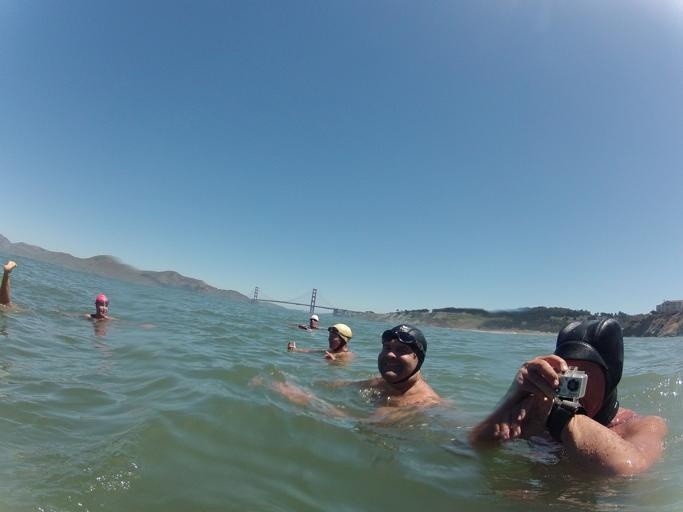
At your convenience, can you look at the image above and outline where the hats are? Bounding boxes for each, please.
[379,323,428,365]
[551,316,626,394]
[328,323,352,343]
[310,314,319,321]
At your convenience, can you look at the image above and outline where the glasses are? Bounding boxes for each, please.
[381,330,415,344]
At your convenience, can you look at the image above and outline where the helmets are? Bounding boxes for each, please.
[94,294,111,307]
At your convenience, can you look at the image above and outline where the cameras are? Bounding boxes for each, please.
[544,372,588,398]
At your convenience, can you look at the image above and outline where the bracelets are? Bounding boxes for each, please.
[544,399,587,441]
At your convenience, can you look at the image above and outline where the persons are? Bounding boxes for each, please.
[464,315,668,482]
[287,322,356,363]
[0,259,18,310]
[52,291,155,329]
[252,323,442,428]
[297,314,321,332]
